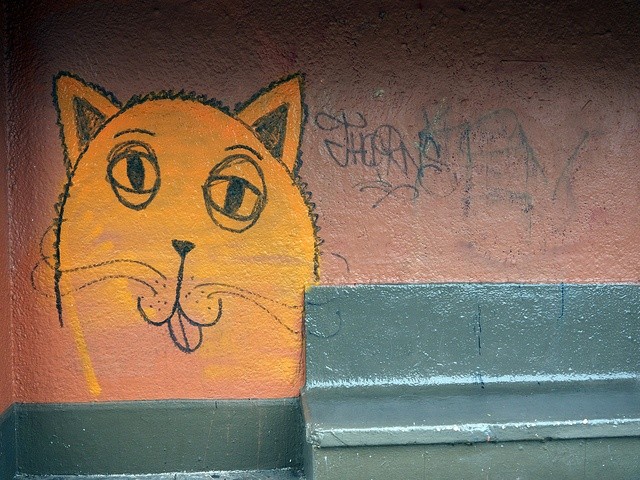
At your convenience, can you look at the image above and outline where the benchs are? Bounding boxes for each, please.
[300,281,639,476]
